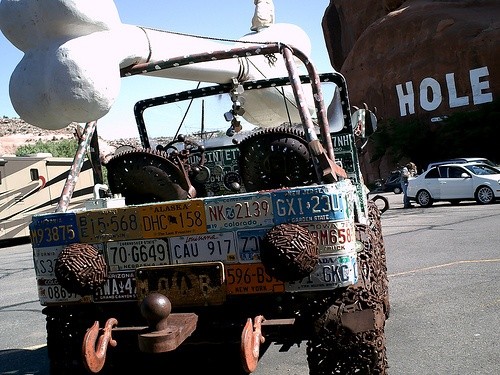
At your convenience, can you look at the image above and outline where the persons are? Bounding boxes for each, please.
[409,165,418,178]
[401,162,415,208]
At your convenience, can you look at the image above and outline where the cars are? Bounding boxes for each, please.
[407,162,500,207]
[384,177,408,194]
[447,158,496,167]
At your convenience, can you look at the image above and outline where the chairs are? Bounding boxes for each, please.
[236,125,325,193]
[106,149,189,205]
